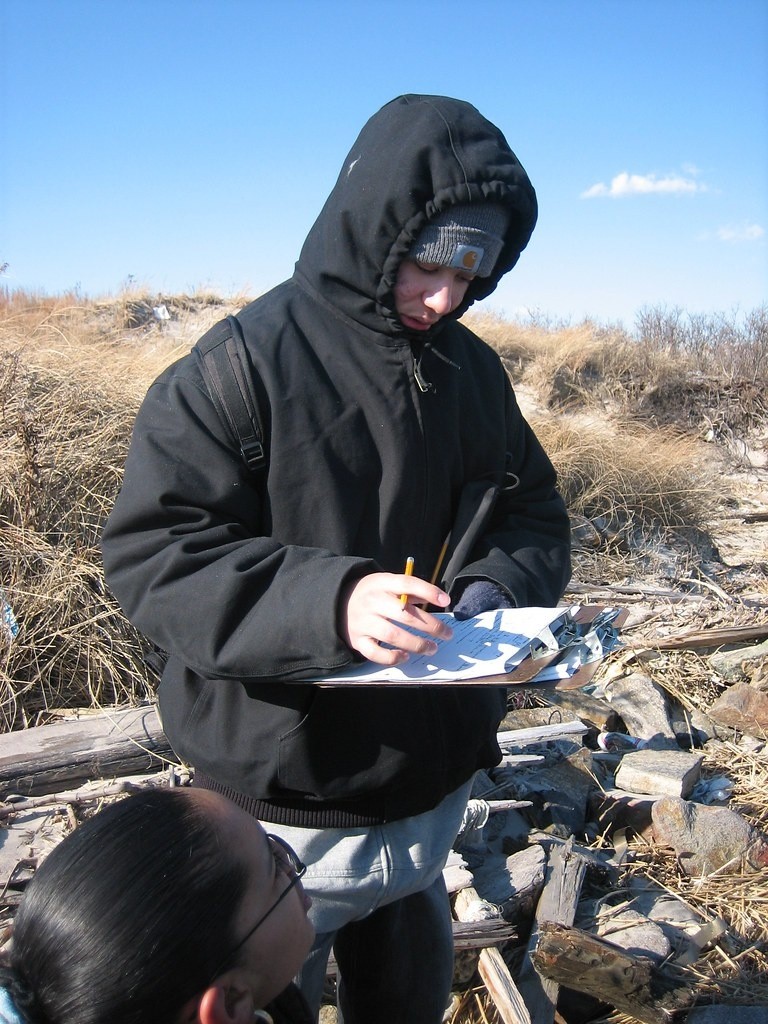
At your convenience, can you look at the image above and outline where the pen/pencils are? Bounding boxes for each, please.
[400,557,414,611]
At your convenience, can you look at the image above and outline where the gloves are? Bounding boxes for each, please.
[453,580,514,621]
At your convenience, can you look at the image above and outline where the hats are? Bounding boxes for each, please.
[408,200,512,278]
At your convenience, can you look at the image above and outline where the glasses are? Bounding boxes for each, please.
[187,832,307,1024]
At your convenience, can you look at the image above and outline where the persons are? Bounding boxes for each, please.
[97,91,576,1023]
[0,787,321,1024]
